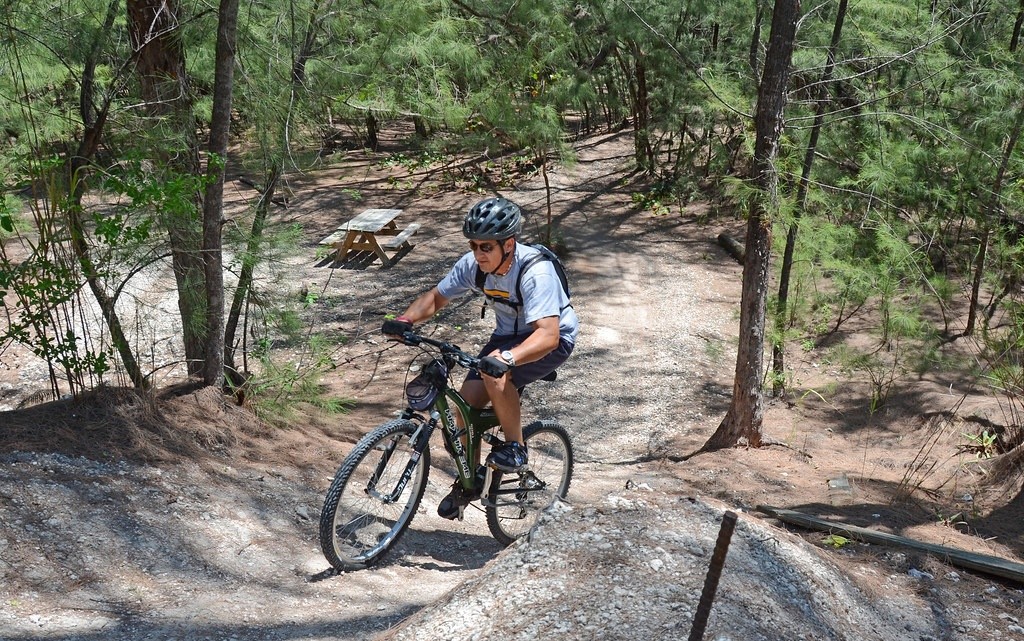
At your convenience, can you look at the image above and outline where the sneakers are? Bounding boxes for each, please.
[438,475,472,519]
[486,441,529,472]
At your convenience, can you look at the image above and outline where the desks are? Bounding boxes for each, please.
[335,209,411,267]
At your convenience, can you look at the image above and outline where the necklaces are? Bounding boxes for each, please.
[502,256,514,276]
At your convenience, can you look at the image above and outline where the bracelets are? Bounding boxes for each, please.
[396,317,413,325]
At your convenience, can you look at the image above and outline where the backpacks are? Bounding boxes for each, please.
[475,242,573,334]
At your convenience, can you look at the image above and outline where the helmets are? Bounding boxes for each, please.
[463,198,521,240]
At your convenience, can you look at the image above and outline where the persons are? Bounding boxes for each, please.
[384,199,580,518]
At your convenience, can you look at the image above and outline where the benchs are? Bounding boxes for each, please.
[318,231,347,246]
[383,223,421,249]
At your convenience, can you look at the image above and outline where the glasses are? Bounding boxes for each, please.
[468,240,498,252]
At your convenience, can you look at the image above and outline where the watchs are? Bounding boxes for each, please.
[497,351,514,366]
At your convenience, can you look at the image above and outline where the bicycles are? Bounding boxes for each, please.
[320,327,576,573]
